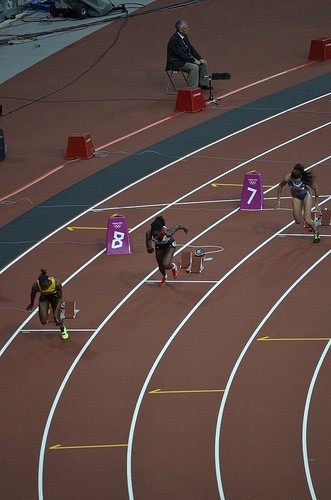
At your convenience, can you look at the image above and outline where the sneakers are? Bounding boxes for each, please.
[160,274,168,287]
[61,325,68,340]
[313,229,320,243]
[171,262,178,279]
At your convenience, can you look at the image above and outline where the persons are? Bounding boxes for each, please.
[167,20,214,90]
[276,164,320,243]
[145,216,188,288]
[26,269,69,339]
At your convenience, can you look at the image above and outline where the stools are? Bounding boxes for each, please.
[165,68,189,95]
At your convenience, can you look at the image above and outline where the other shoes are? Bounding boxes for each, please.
[198,84,213,90]
